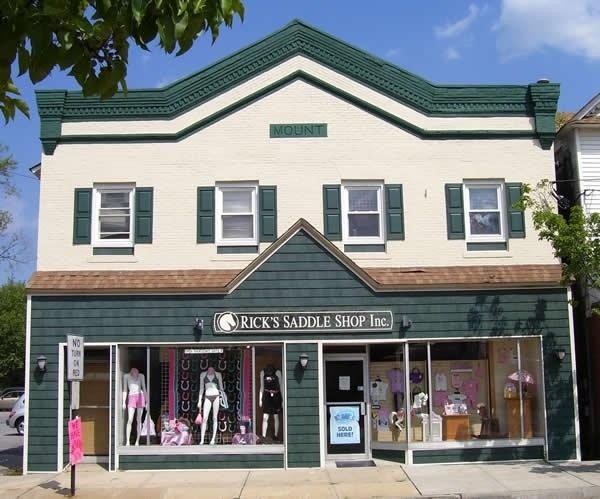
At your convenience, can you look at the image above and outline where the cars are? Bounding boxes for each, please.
[0,387,25,435]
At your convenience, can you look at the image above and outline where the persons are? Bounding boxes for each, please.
[259,366,283,438]
[197,367,229,445]
[122,368,148,446]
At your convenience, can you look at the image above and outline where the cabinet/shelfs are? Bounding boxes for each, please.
[442,414,471,440]
[504,396,534,438]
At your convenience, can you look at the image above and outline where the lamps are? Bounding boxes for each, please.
[552,345,566,361]
[298,353,310,369]
[37,354,48,372]
